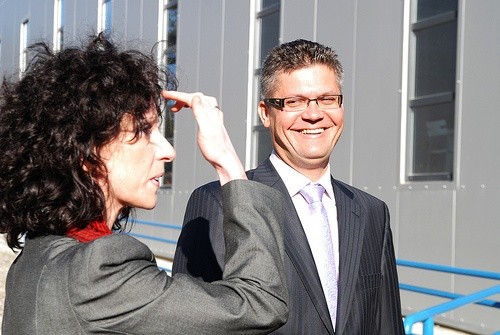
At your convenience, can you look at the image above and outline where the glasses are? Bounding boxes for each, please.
[263,95,343,111]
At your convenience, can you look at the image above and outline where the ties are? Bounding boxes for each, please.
[300,185,337,332]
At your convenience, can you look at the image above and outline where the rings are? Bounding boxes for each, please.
[215,106,218,108]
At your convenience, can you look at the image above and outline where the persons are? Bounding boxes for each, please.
[171,39,406,335]
[0,30,289,335]
